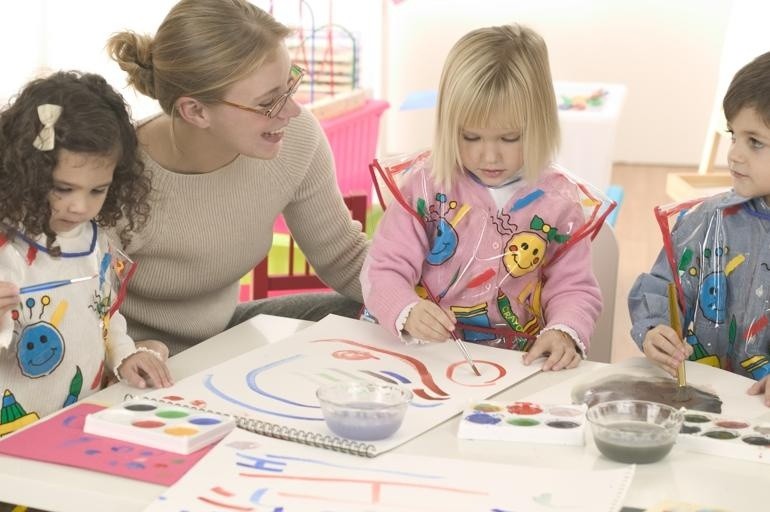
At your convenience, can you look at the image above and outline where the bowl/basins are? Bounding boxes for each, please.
[316,380,414,441]
[585,399,685,464]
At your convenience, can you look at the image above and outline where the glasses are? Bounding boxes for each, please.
[215,64,305,120]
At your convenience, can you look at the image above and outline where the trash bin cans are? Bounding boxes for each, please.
[535,80,621,212]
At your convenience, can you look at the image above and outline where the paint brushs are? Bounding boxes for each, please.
[421,278,482,378]
[19,274,101,296]
[669,283,694,400]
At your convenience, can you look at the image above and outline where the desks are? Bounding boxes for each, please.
[0,309,769,510]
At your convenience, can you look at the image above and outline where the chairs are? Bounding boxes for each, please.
[247,98,390,306]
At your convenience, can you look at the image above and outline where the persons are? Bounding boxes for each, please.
[627,51,770,408]
[0,70,174,438]
[358,21,617,371]
[105,1,371,358]
